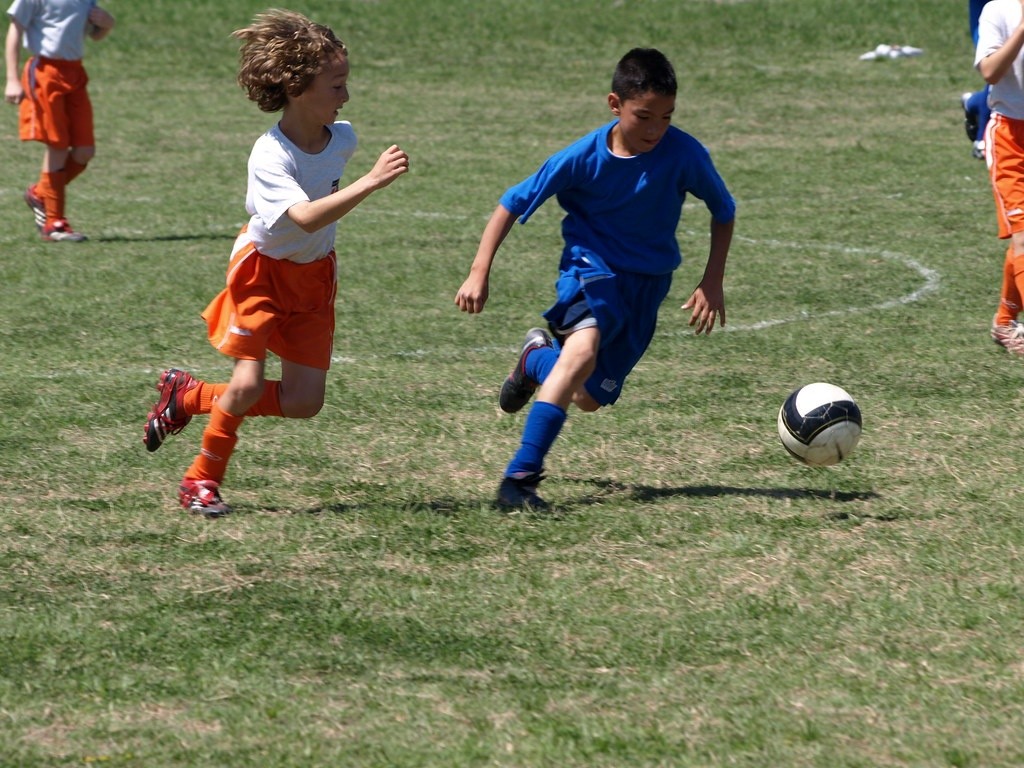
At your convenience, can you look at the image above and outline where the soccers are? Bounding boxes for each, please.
[776,381,863,469]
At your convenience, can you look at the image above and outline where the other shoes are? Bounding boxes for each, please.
[972,140,986,159]
[961,92,979,142]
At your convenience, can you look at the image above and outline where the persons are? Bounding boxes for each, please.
[5,1,115,241]
[142,9,410,515]
[452,49,737,512]
[960,0,1024,357]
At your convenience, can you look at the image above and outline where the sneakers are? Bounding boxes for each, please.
[42,217,87,244]
[991,313,1024,358]
[491,474,552,513]
[144,368,199,452]
[500,326,554,413]
[179,476,233,517]
[22,184,47,232]
